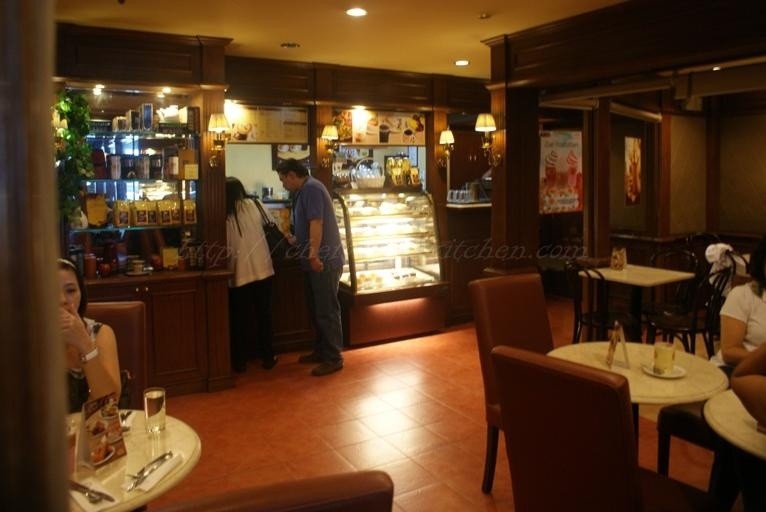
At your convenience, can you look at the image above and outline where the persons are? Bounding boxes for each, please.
[57,258,122,414]
[275,157,346,376]
[225,176,284,373]
[730,340,766,427]
[708,241,766,390]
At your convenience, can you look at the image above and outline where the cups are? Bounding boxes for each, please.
[380,125,391,142]
[567,164,578,186]
[142,386,165,432]
[545,166,557,189]
[261,185,273,202]
[653,337,676,373]
[403,128,417,145]
[273,192,290,202]
[131,259,146,273]
[445,188,475,203]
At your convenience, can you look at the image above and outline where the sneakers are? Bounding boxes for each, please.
[312,361,343,376]
[299,354,322,364]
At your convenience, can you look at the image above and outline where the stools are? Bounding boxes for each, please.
[653,396,718,483]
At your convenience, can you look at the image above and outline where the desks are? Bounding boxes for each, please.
[55,405,205,512]
[702,387,765,465]
[585,259,698,344]
[543,340,728,448]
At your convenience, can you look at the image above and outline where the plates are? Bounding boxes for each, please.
[89,401,121,467]
[126,270,148,277]
[382,115,408,133]
[638,363,688,379]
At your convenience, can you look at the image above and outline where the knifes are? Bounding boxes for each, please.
[68,478,115,501]
[125,453,173,492]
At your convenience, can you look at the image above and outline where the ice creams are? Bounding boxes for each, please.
[545,150,559,183]
[566,149,579,185]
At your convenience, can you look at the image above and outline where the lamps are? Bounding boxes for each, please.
[433,128,459,174]
[203,111,231,170]
[315,123,340,175]
[472,111,504,170]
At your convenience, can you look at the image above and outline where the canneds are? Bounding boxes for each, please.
[132,260,144,273]
[84,253,97,280]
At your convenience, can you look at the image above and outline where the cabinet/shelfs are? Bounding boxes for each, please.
[326,187,452,353]
[69,122,201,232]
[77,264,232,393]
[256,201,319,352]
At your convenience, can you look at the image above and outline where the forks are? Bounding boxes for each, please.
[127,451,170,478]
[71,484,103,504]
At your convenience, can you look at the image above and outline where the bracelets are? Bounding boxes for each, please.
[81,348,98,363]
[308,255,316,263]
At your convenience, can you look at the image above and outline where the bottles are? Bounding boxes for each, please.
[610,246,628,272]
[108,142,179,181]
[69,246,96,279]
[178,228,203,272]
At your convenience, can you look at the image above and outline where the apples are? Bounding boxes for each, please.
[98,264,111,278]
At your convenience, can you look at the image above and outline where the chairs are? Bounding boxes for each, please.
[79,296,155,412]
[566,259,633,341]
[145,467,394,512]
[488,339,709,511]
[465,271,556,496]
[633,229,753,363]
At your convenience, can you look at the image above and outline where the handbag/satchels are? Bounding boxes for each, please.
[250,196,298,276]
